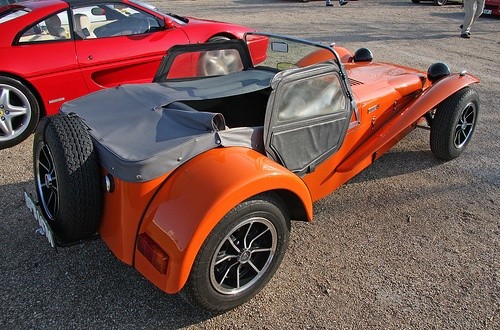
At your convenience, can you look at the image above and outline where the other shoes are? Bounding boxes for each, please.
[459,25,471,35]
[460,33,470,39]
[325,4,333,6]
[340,1,348,5]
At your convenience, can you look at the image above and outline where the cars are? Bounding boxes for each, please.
[412,0,500,19]
[0,0,268,148]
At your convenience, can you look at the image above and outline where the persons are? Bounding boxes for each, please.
[459,0,485,39]
[326,0,348,6]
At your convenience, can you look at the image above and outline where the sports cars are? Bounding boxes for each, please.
[28,31,480,315]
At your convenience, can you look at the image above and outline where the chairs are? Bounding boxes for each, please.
[74,13,97,39]
[45,14,66,38]
